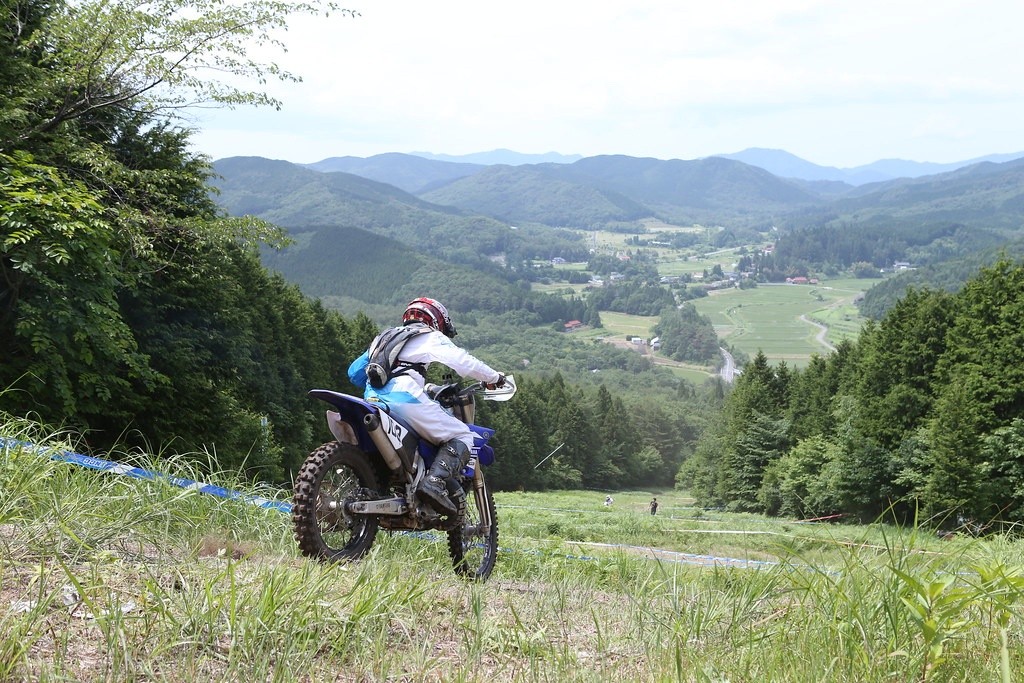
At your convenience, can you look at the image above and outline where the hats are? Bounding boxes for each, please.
[606,495,610,499]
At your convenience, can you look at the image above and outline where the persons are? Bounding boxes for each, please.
[348,297,505,515]
[604,496,613,506]
[648,498,658,515]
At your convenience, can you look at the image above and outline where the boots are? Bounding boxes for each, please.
[416,438,470,516]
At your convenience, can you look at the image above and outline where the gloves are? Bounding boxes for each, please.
[486,373,505,390]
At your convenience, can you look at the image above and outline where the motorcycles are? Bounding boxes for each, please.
[291,369,516,583]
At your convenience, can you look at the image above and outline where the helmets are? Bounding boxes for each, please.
[403,297,457,338]
